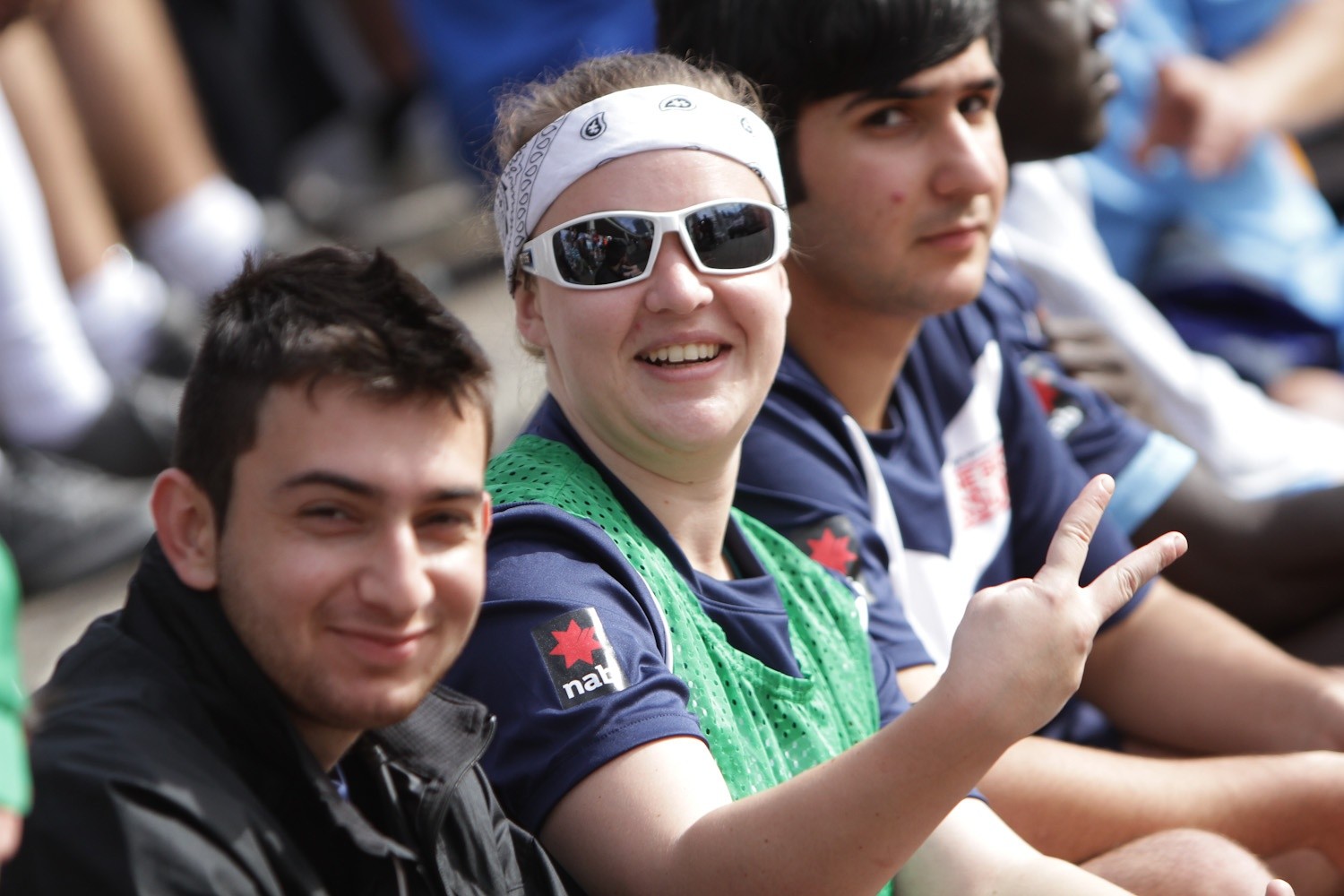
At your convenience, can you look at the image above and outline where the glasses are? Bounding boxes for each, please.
[514,200,792,290]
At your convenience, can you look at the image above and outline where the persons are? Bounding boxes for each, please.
[0,247,571,896]
[651,0,1344,896]
[0,0,1344,896]
[428,47,1187,896]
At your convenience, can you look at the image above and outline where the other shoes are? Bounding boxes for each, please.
[131,284,212,375]
[68,368,198,476]
[1,435,161,592]
